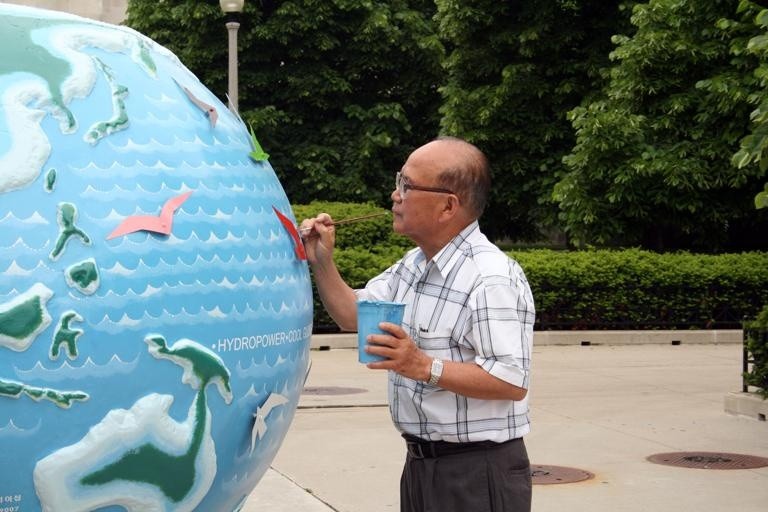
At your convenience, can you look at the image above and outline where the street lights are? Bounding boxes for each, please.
[217,0,245,112]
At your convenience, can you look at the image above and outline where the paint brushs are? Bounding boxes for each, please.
[297,213,386,232]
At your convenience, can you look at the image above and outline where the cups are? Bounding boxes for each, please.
[355,300,407,365]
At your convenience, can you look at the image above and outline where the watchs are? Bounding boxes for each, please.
[424,357,445,386]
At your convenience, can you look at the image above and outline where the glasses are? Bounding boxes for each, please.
[395,171,455,200]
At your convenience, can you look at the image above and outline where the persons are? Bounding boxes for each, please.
[299,135,537,511]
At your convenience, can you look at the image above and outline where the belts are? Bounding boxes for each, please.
[405,439,516,459]
